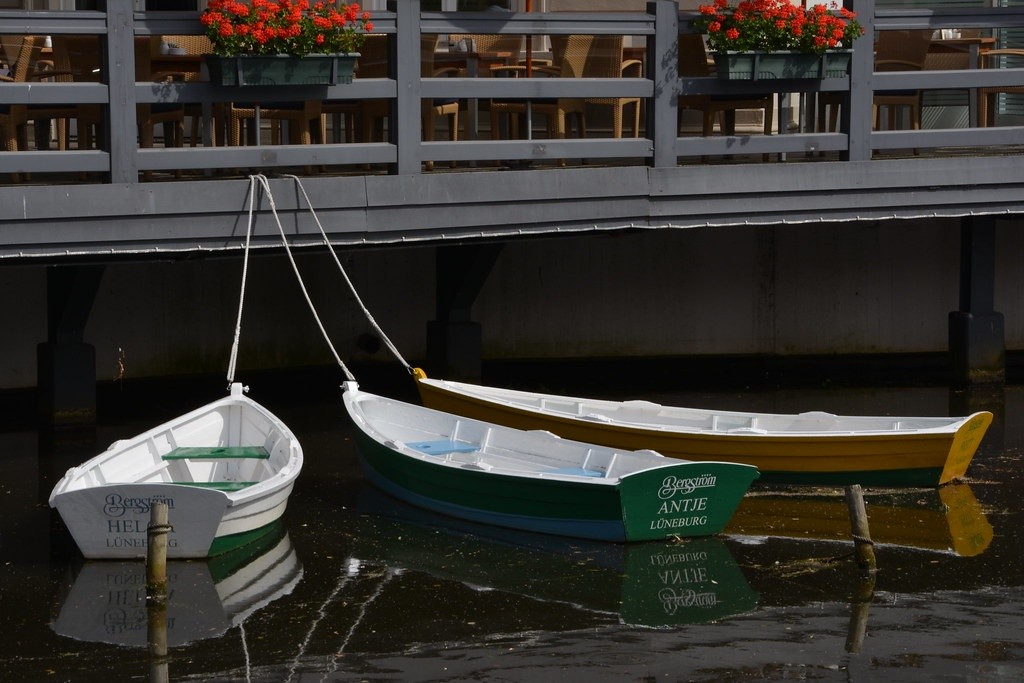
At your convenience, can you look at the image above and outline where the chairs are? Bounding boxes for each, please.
[0,30,1024,183]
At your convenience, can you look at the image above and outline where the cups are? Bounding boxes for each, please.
[160,42,170,54]
[941,28,952,39]
[952,29,961,39]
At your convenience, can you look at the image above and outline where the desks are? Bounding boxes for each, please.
[927,37,999,128]
[152,53,216,178]
[436,52,513,167]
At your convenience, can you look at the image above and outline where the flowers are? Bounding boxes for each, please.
[199,0,374,57]
[685,0,868,53]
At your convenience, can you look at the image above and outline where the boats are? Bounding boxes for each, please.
[415,367,994,485]
[49,380,305,557]
[721,472,995,559]
[346,501,761,629]
[53,514,306,647]
[342,379,761,544]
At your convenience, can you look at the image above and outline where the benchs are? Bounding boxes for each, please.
[540,466,605,477]
[161,447,268,461]
[168,482,259,491]
[405,441,478,455]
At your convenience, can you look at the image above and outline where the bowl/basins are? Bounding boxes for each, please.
[170,48,185,54]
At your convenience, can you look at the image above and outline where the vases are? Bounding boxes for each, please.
[707,47,856,81]
[216,52,362,86]
